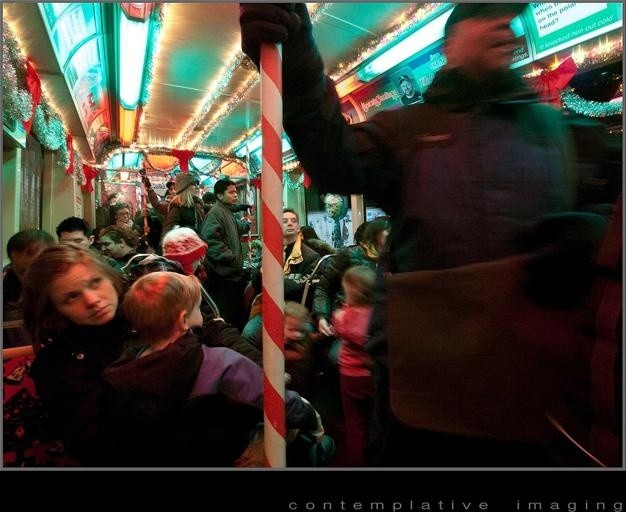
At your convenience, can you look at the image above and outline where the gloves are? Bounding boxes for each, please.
[238,2,329,84]
[510,203,616,314]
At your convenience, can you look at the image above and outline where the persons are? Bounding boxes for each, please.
[0,168,393,468]
[238,2,625,469]
[398,74,422,106]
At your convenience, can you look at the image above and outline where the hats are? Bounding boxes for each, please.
[399,74,411,83]
[444,3,529,28]
[176,173,199,194]
[162,224,208,278]
[166,177,176,187]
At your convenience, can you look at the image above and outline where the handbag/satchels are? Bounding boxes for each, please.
[377,249,623,451]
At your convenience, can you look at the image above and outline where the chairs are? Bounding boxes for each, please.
[301,253,338,313]
